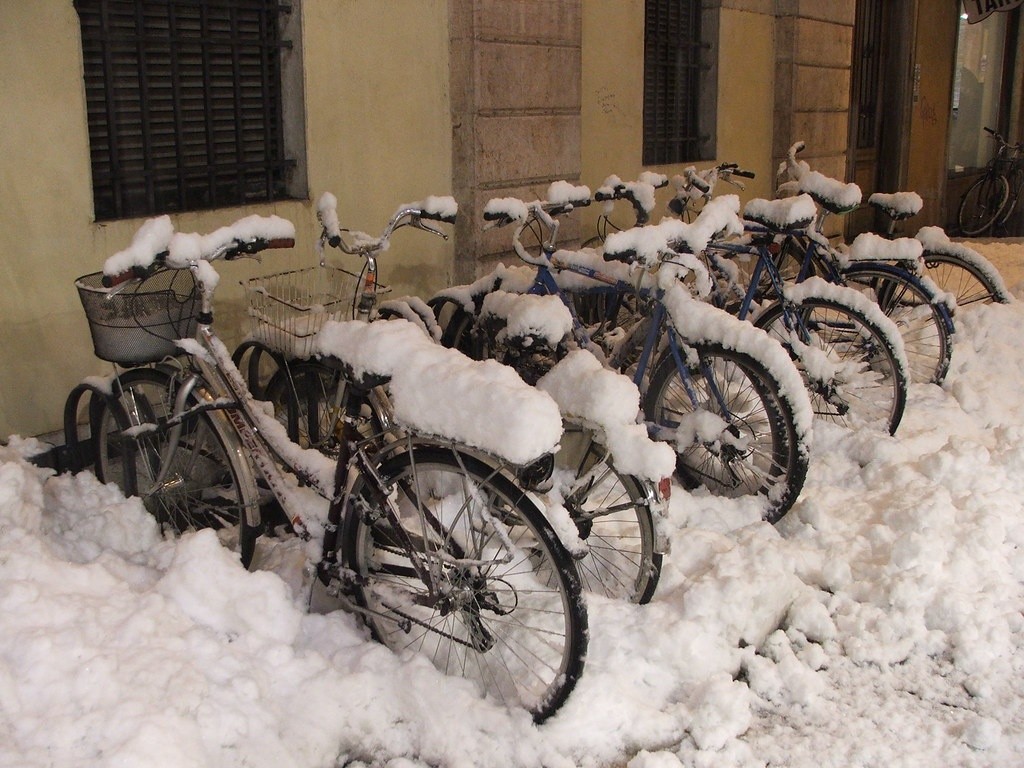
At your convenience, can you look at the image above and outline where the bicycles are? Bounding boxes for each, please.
[262,205,674,607]
[73,233,588,725]
[957,126,1024,238]
[413,136,1010,528]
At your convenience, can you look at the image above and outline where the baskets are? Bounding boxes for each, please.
[238,264,391,362]
[73,266,202,366]
